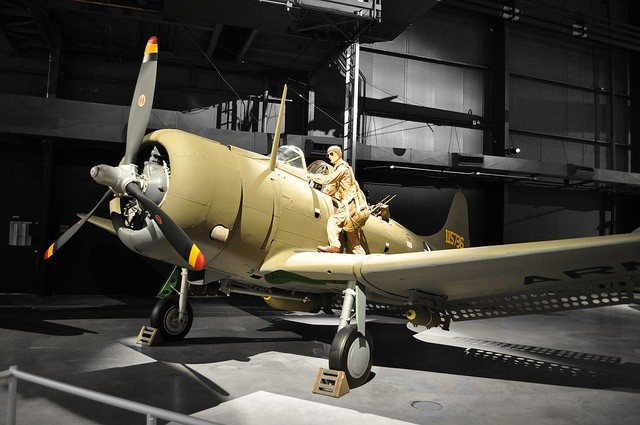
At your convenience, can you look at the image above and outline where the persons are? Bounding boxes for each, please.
[307,145,370,255]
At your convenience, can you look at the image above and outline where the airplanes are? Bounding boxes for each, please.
[43,35,640,388]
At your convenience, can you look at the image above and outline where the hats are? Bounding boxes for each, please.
[327,146,342,159]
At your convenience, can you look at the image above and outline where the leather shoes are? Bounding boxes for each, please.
[317,245,340,252]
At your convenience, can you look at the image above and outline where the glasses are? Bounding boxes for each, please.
[322,167,328,169]
[327,153,333,157]
